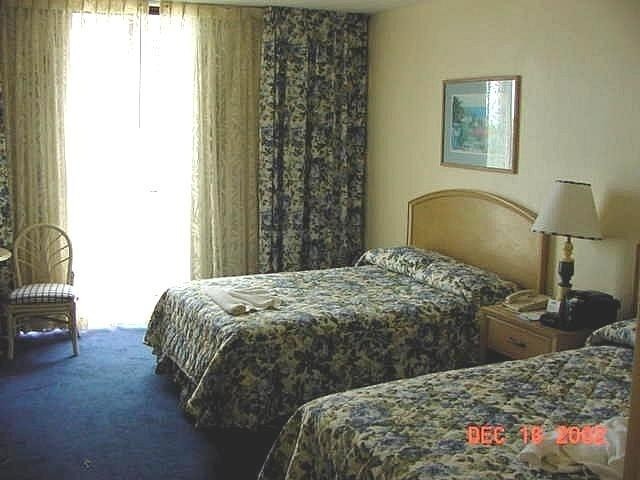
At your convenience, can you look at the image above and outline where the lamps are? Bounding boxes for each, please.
[531,178,604,312]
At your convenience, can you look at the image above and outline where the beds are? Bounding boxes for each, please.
[143,190,550,433]
[257,244,640,480]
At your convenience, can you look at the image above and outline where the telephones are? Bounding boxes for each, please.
[502,288,549,312]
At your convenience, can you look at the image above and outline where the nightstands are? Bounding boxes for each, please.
[480,304,593,366]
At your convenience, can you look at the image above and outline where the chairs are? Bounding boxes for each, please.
[8,224,80,361]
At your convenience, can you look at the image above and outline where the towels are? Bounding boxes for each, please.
[585,317,635,347]
[517,415,629,480]
[355,245,457,278]
[208,286,281,315]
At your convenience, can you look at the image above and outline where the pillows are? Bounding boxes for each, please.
[413,261,521,300]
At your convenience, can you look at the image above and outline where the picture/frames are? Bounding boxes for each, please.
[440,75,520,174]
